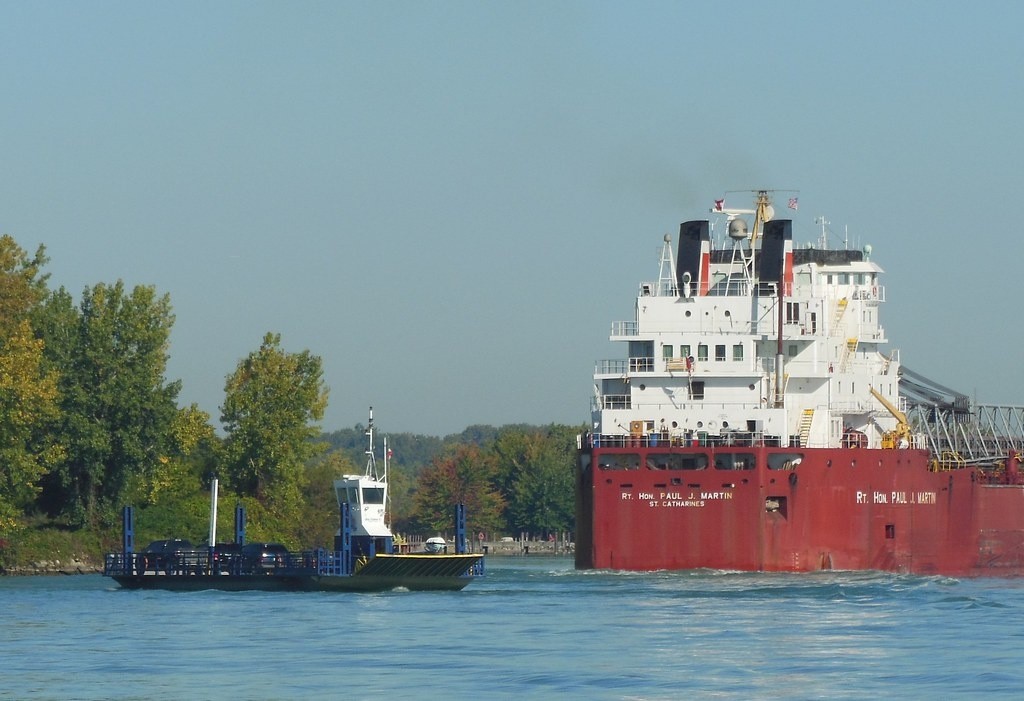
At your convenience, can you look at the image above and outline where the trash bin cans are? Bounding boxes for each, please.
[790,436,800,448]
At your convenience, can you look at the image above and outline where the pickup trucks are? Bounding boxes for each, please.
[135,539,207,574]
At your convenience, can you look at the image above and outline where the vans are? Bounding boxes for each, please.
[499,537,513,542]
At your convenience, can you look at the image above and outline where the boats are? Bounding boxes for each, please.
[574,188,1024,582]
[425,537,445,550]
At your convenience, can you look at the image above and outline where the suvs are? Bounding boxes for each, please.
[229,543,291,576]
[188,543,234,576]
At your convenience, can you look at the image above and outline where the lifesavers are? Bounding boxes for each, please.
[135,554,148,568]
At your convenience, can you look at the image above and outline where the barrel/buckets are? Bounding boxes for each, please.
[593,433,661,448]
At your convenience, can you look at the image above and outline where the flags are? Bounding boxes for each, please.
[788,197,798,210]
[714,198,725,212]
